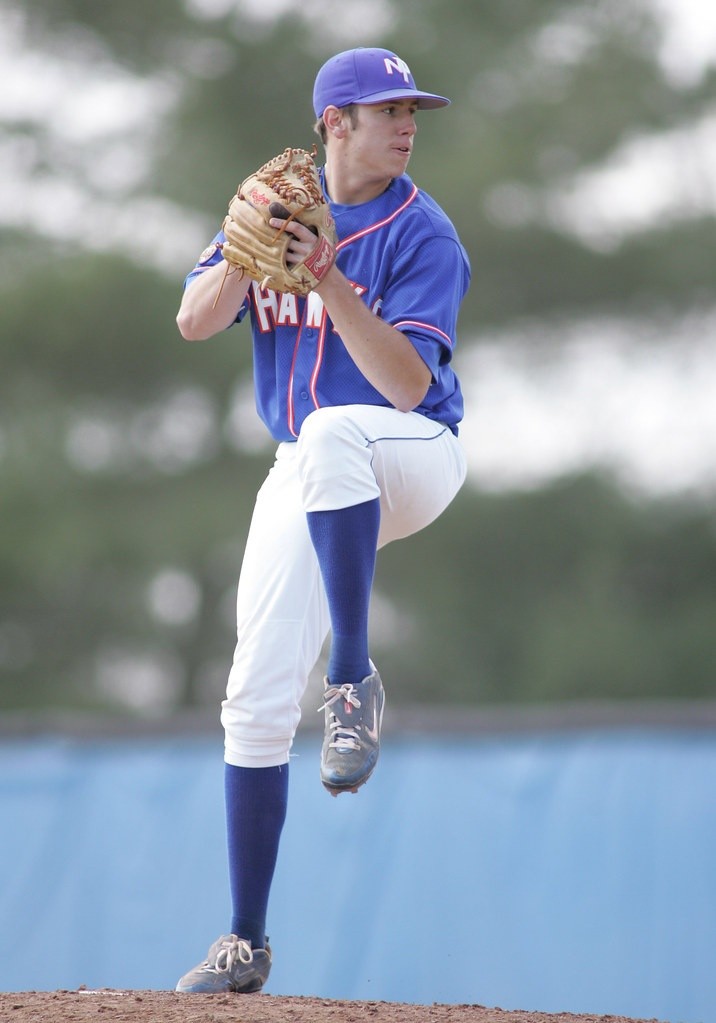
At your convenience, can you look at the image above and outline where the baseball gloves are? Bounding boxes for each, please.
[214,143,339,300]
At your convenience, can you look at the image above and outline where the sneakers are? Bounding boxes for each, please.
[176,935,273,997]
[316,657,385,795]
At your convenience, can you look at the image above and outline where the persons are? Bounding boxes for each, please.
[174,48,470,994]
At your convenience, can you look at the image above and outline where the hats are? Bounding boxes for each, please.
[312,48,450,120]
